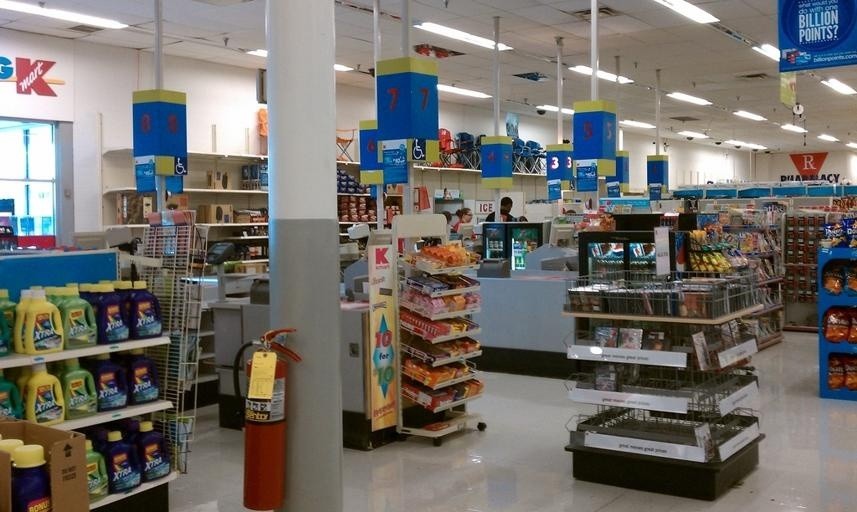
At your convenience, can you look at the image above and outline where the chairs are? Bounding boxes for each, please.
[332,127,357,162]
[427,128,547,174]
[254,110,267,153]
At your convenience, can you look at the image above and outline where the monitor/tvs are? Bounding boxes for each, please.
[364,230,392,252]
[458,223,473,236]
[550,224,574,244]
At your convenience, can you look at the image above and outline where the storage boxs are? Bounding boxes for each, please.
[162,195,190,212]
[205,168,231,191]
[0,417,94,512]
[199,202,235,225]
[235,262,268,273]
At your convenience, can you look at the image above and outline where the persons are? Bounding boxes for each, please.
[454,208,475,240]
[442,187,452,198]
[438,210,457,234]
[485,196,517,222]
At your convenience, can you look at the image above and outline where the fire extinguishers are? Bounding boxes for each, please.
[233,329,302,510]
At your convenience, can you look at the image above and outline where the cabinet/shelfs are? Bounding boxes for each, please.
[97,145,403,412]
[677,229,736,283]
[393,215,487,448]
[673,185,857,209]
[0,250,179,512]
[700,210,787,352]
[410,161,552,226]
[816,244,857,404]
[778,204,857,333]
[563,272,765,502]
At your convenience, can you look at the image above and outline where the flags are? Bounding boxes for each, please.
[777,71,796,108]
[777,0,857,75]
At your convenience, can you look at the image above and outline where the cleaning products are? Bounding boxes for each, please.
[1,421,173,512]
[1,346,174,425]
[1,278,173,357]
